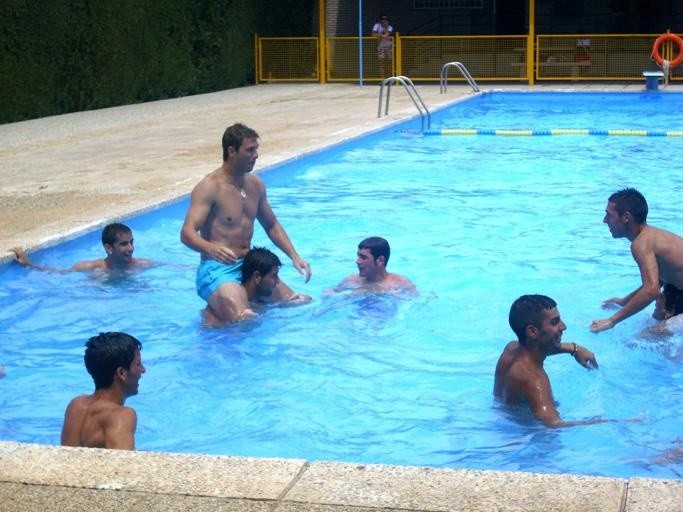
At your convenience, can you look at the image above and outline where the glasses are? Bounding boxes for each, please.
[380,18,388,20]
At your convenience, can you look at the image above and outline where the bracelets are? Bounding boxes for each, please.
[570,341,579,357]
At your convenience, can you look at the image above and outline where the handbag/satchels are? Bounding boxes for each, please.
[520,50,566,62]
[575,54,592,62]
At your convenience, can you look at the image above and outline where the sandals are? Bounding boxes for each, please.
[378,81,383,85]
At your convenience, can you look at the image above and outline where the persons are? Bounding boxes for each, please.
[590,183,681,337]
[199,244,315,327]
[371,12,394,86]
[629,284,682,363]
[4,222,196,282]
[321,235,420,299]
[58,332,147,452]
[179,121,310,325]
[621,442,682,470]
[491,293,642,432]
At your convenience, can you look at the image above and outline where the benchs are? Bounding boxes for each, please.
[510,47,592,77]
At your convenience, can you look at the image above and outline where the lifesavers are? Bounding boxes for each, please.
[652,34,683,68]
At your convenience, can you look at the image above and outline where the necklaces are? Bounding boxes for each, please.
[219,165,250,199]
